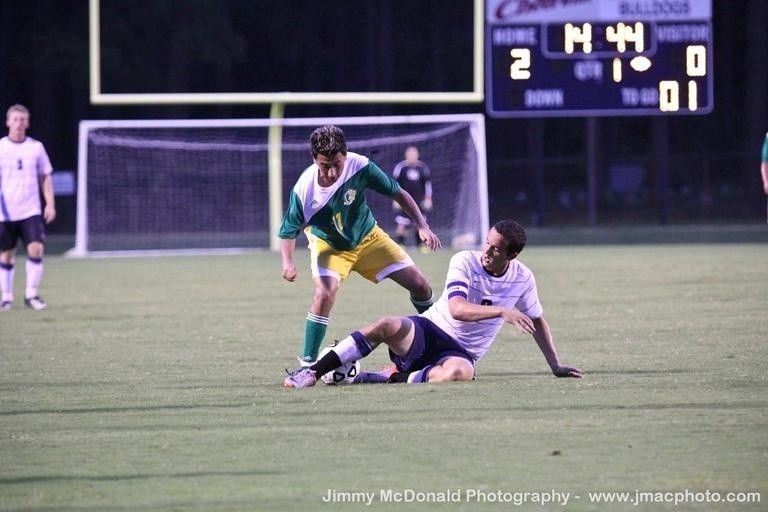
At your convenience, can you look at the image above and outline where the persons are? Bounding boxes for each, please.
[390,147,434,255]
[760,131,768,195]
[0,104,56,311]
[283,220,582,389]
[277,124,443,371]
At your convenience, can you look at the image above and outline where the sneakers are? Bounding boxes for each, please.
[284,369,315,388]
[354,370,369,384]
[1,298,49,314]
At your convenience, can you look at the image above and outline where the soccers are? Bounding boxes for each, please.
[315,344,360,386]
